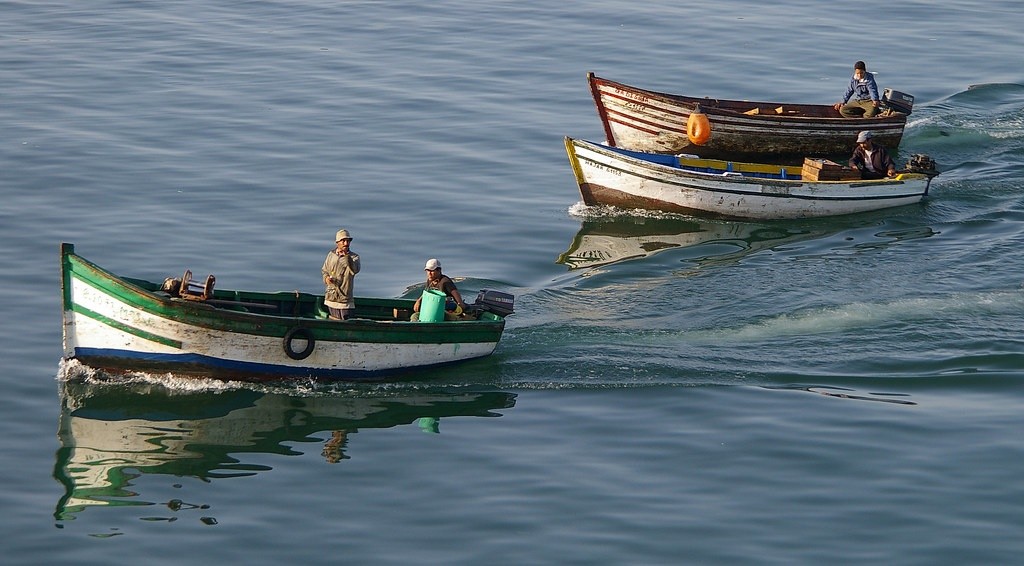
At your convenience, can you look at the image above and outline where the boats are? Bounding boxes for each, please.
[59,243,506,375]
[586,72,906,164]
[563,135,929,225]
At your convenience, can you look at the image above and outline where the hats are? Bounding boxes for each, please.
[424,259,442,271]
[857,131,872,142]
[335,229,353,243]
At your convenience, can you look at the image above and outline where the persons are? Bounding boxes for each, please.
[849,131,895,178]
[833,60,879,118]
[322,230,360,320]
[410,258,465,324]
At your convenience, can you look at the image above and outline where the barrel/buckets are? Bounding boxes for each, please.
[419,288,447,322]
